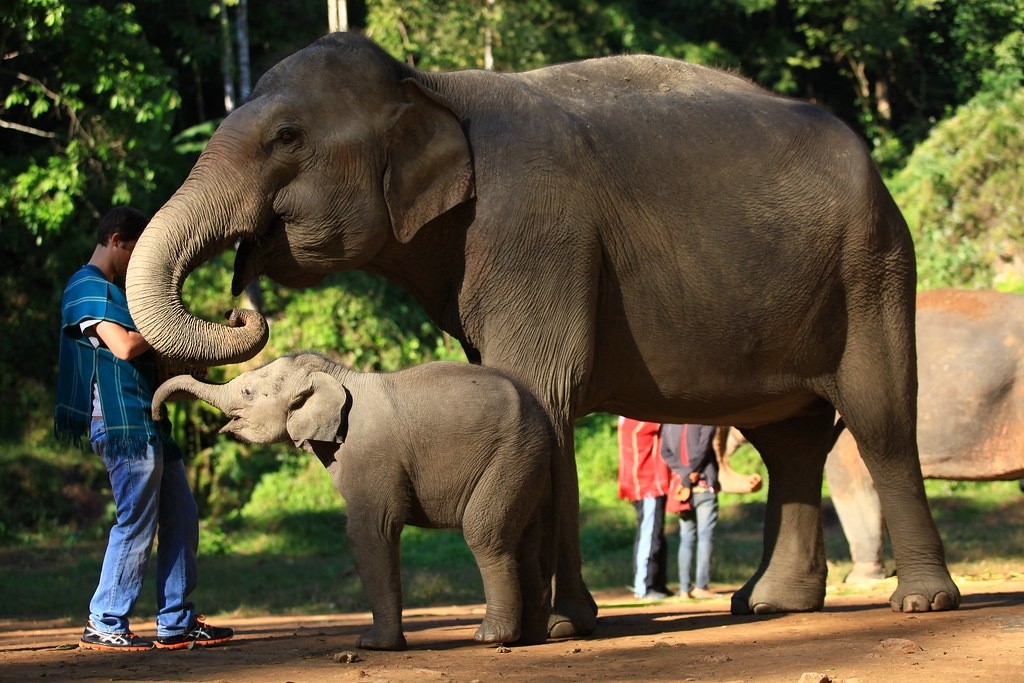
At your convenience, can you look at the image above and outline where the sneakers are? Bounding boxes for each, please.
[154,612,233,649]
[79,616,154,651]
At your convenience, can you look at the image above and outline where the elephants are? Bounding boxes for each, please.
[124,31,961,634]
[716,284,1023,583]
[149,352,559,653]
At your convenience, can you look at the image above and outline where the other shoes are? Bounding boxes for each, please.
[634,585,674,601]
[678,586,720,600]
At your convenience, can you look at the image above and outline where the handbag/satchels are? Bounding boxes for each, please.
[666,471,692,514]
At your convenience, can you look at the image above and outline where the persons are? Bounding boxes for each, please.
[617,416,723,599]
[61,200,234,651]
